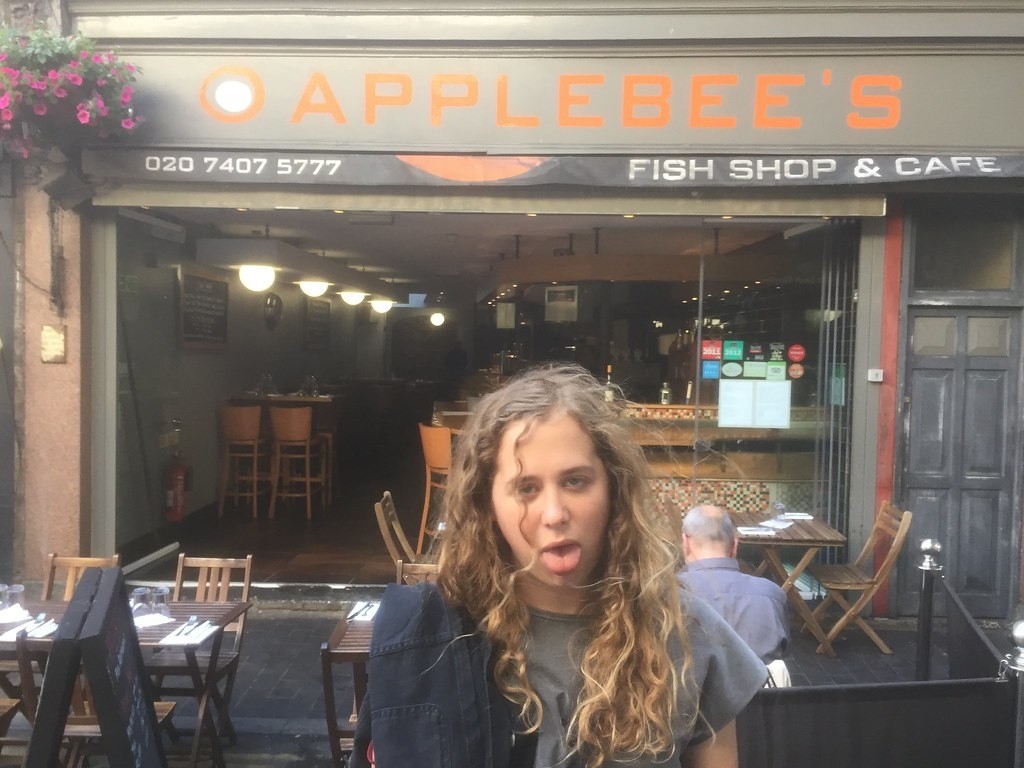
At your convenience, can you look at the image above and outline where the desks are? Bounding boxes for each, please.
[0,601,253,768]
[219,392,332,402]
[729,511,848,659]
[321,600,381,718]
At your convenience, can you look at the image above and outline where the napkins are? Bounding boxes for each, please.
[737,527,775,536]
[158,622,220,646]
[778,512,813,520]
[0,618,59,642]
[347,601,381,621]
[133,613,176,628]
[0,602,33,624]
[759,518,794,529]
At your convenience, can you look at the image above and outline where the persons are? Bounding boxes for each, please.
[676,505,791,665]
[348,364,769,768]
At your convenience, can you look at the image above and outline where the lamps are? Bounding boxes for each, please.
[196,239,409,315]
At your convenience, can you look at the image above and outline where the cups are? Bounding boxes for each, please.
[658,381,672,405]
[7,584,25,610]
[0,584,9,610]
[150,588,170,619]
[131,587,151,617]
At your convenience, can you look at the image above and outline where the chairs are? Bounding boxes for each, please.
[16,629,176,768]
[267,405,327,520]
[374,491,434,585]
[0,552,125,725]
[416,422,453,554]
[320,641,374,768]
[216,406,262,519]
[800,500,913,658]
[143,552,254,768]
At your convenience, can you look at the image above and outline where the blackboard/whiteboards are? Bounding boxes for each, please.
[182,273,228,341]
[24,566,169,768]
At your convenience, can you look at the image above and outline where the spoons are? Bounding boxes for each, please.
[175,615,197,636]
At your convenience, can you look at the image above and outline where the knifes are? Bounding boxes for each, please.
[348,603,370,619]
[362,604,374,616]
[27,619,55,633]
[185,620,206,635]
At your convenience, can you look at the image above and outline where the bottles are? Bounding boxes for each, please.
[602,364,615,403]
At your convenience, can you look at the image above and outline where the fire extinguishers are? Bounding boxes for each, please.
[162,448,187,523]
